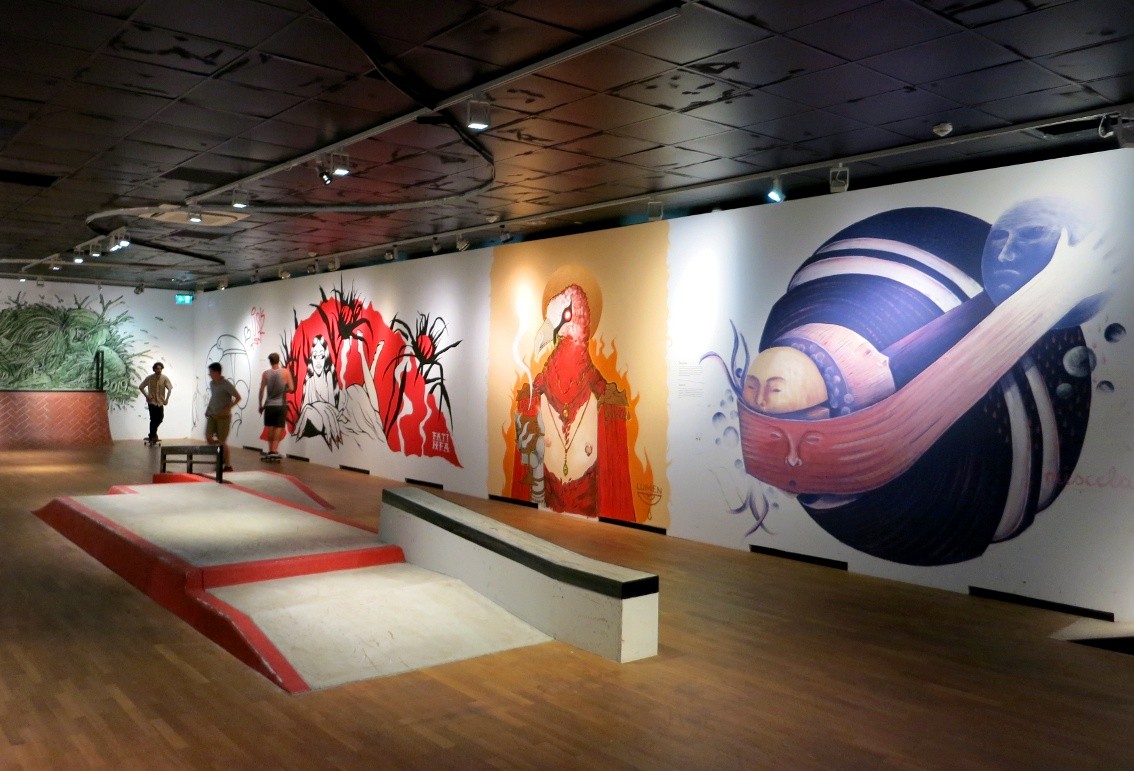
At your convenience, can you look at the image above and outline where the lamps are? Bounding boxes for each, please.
[314,149,351,185]
[455,232,471,252]
[499,225,513,244]
[187,201,202,223]
[20,226,129,283]
[1110,113,1134,149]
[232,184,250,208]
[432,237,442,253]
[646,200,664,222]
[465,93,489,130]
[830,162,850,193]
[384,250,394,261]
[767,174,786,203]
[197,255,342,292]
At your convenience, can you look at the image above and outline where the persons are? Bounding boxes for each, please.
[138,362,173,444]
[204,362,241,473]
[258,352,294,458]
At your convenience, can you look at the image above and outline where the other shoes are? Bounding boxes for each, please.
[266,451,279,457]
[223,466,234,472]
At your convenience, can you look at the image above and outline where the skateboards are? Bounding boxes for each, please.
[258,452,282,463]
[143,437,162,447]
